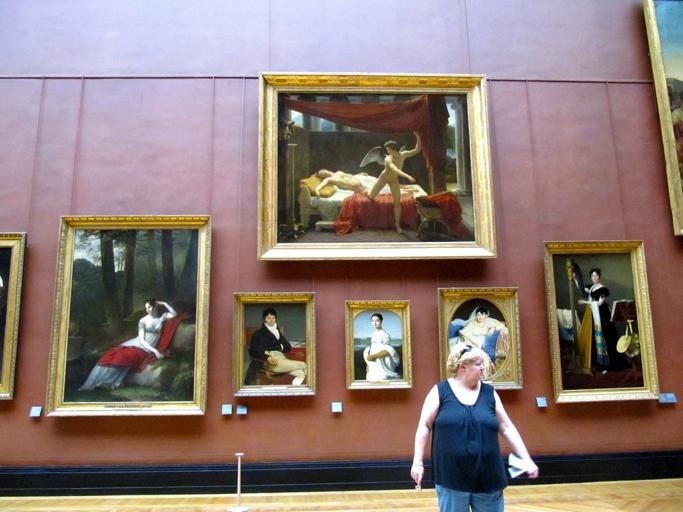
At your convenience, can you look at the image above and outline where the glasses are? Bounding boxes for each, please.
[458,345,471,361]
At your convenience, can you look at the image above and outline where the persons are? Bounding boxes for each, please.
[572,267,615,374]
[409,341,540,512]
[78,298,177,391]
[362,312,402,381]
[456,305,505,378]
[315,167,418,199]
[249,307,308,386]
[365,130,423,232]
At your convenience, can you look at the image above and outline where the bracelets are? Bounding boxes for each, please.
[413,464,424,467]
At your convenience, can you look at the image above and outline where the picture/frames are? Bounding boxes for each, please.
[641,0,683,237]
[255,72,498,261]
[231,292,316,398]
[542,241,661,405]
[44,214,212,417]
[344,300,413,391]
[0,232,27,400]
[437,286,524,391]
